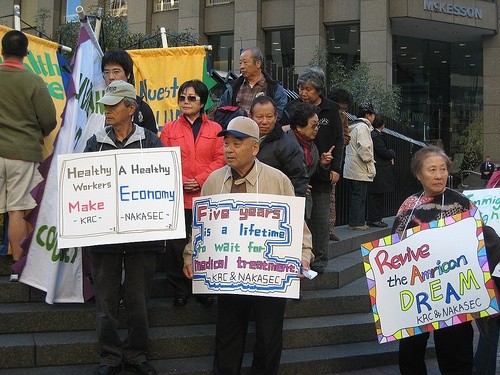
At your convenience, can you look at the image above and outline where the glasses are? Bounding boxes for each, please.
[102,70,124,78]
[307,122,321,130]
[179,95,200,101]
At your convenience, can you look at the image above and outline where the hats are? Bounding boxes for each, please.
[217,116,259,140]
[96,80,136,105]
[358,102,378,114]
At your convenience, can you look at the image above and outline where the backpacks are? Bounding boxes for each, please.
[211,106,248,130]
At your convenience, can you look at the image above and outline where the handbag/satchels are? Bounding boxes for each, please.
[483,226,500,273]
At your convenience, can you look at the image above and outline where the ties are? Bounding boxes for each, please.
[487,162,488,170]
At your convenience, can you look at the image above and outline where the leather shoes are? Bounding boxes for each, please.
[94,364,122,375]
[123,362,156,375]
[195,295,215,304]
[174,297,185,306]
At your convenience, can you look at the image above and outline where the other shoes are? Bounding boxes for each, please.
[364,224,369,229]
[9,274,18,282]
[311,266,322,274]
[366,221,385,227]
[349,226,367,231]
[329,234,339,241]
[380,221,388,227]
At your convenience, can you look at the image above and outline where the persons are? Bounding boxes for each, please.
[0,29,57,280]
[159,81,227,310]
[81,80,162,375]
[283,65,342,276]
[342,103,388,230]
[222,47,286,126]
[101,48,159,137]
[391,146,483,375]
[479,156,494,189]
[326,89,353,240]
[183,117,314,375]
[250,96,312,198]
